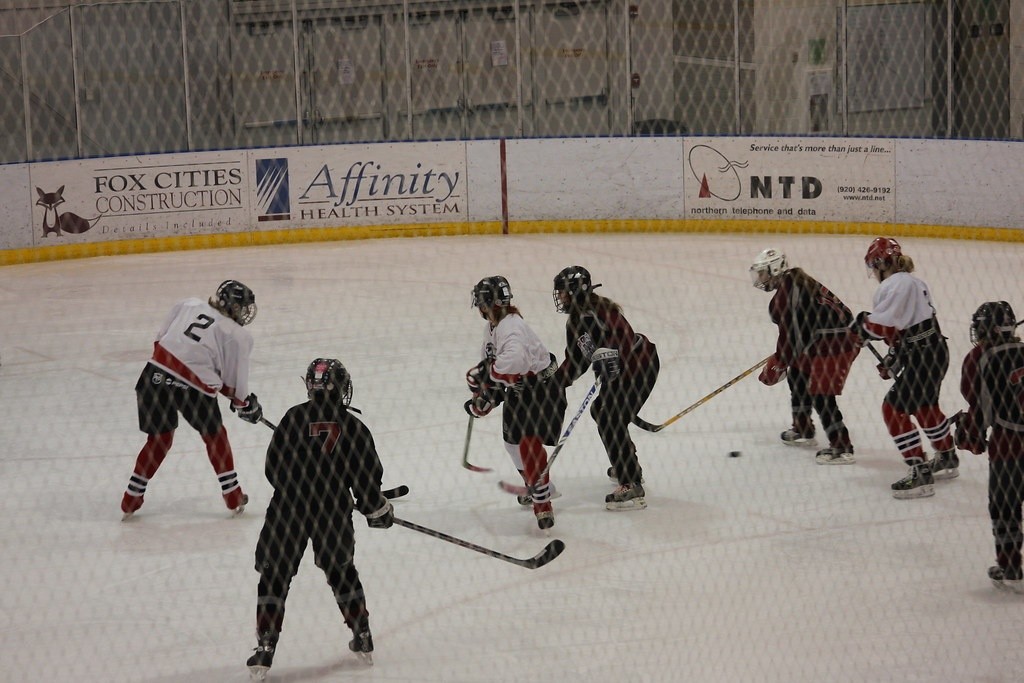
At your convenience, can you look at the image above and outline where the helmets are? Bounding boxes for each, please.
[553,265,592,313]
[218,279,257,326]
[472,275,511,319]
[864,236,901,275]
[970,300,1016,351]
[750,248,789,292]
[305,357,353,407]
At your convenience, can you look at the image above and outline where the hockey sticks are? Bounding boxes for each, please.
[353,503,566,570]
[259,415,409,500]
[461,390,494,473]
[497,373,602,498]
[630,352,774,433]
[866,342,963,425]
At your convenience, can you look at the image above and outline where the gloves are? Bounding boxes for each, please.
[359,495,394,528]
[464,391,501,418]
[237,392,264,424]
[590,346,620,384]
[877,347,902,380]
[847,311,884,348]
[466,357,495,392]
[954,414,987,455]
[759,355,787,386]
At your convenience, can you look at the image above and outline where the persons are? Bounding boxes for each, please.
[553,265,660,511]
[246,358,394,683]
[849,237,960,499]
[749,248,860,465]
[955,301,1024,595]
[120,280,263,522]
[464,276,567,534]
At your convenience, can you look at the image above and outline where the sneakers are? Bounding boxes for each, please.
[518,482,559,506]
[607,464,645,483]
[987,564,1024,593]
[348,622,375,665]
[533,502,555,536]
[246,634,277,681]
[120,500,143,521]
[605,482,647,511]
[226,494,248,518]
[782,420,959,498]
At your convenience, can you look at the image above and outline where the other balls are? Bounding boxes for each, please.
[729,451,741,457]
